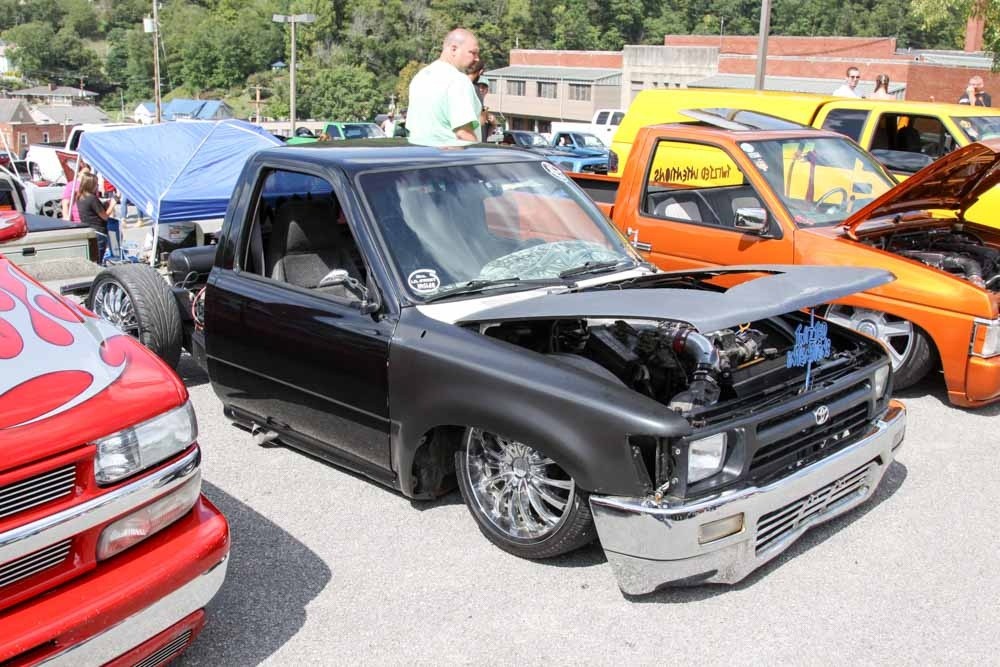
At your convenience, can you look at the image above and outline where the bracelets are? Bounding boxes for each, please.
[113,196,120,204]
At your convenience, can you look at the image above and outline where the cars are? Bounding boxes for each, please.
[0,207,231,667]
[198,138,909,596]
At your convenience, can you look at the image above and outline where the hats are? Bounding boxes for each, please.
[477,75,489,86]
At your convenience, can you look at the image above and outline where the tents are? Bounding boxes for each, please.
[67,118,335,268]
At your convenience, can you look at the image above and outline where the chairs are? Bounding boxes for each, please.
[152,221,205,268]
[266,199,365,299]
[896,126,921,152]
[713,185,780,236]
[611,115,620,124]
[647,190,718,225]
[103,239,141,267]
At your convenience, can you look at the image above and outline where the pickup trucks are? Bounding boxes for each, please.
[268,86,1000,412]
[26,121,141,183]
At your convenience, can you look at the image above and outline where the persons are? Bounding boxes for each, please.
[955,75,990,107]
[960,118,982,140]
[558,137,566,147]
[833,66,895,100]
[120,191,143,226]
[61,162,121,267]
[464,56,497,145]
[152,222,197,264]
[405,27,483,145]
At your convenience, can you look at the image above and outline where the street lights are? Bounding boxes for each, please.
[271,11,315,139]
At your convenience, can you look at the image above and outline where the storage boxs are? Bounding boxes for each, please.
[0,239,90,265]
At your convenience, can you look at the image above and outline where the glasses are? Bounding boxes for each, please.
[849,76,859,79]
